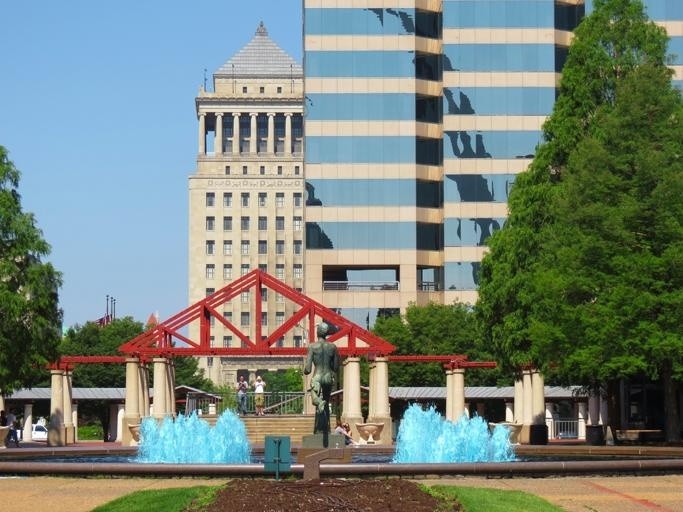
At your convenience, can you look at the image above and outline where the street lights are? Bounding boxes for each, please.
[105,294,116,320]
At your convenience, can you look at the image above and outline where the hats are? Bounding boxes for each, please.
[256,375,262,382]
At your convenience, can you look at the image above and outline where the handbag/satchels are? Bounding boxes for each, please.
[239,382,247,394]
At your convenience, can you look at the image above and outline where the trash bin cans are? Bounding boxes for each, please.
[586,425,603,445]
[530,425,548,445]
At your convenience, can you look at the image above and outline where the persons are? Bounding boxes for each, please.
[4,406,22,448]
[0,410,7,426]
[335,423,353,440]
[251,375,266,416]
[302,321,340,434]
[342,422,353,445]
[235,374,249,416]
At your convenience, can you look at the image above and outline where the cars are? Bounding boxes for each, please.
[10,424,48,442]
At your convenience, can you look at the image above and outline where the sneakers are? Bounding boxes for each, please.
[236,410,265,417]
